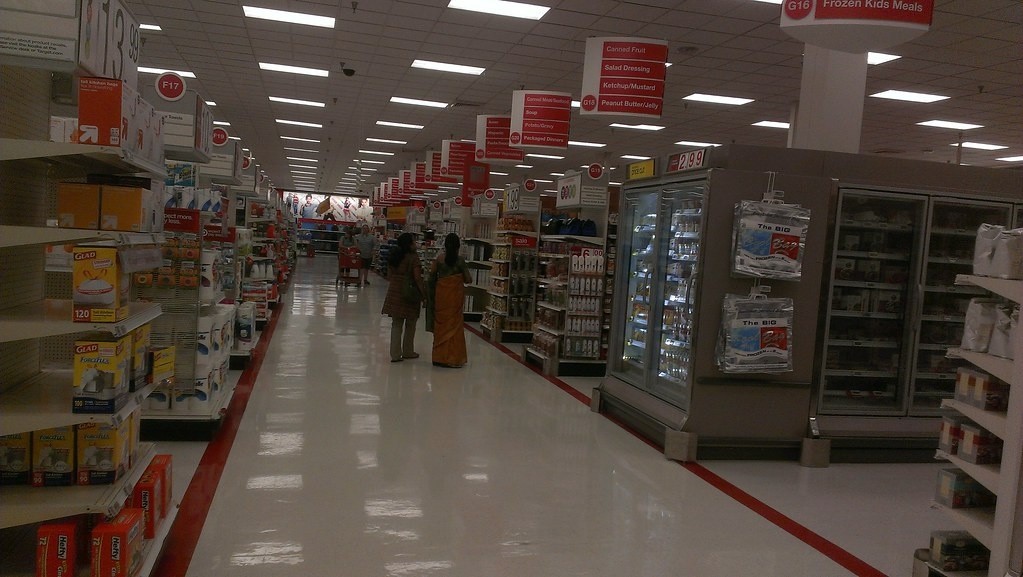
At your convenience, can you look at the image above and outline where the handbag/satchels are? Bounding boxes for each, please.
[402,256,421,302]
[545,217,597,237]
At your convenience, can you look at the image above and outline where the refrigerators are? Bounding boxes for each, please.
[602,165,838,453]
[817,182,1023,417]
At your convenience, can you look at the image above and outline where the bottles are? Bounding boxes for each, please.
[566,254,602,358]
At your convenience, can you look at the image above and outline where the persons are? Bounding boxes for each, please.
[293,193,299,216]
[381,232,427,363]
[285,193,293,214]
[430,233,473,369]
[300,195,318,218]
[323,194,336,221]
[342,197,373,222]
[354,225,376,287]
[338,231,358,287]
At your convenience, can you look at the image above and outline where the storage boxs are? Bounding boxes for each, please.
[36,453,173,577]
[938,415,1002,466]
[142,184,269,414]
[936,467,995,509]
[57,175,167,233]
[930,529,989,570]
[79,76,165,164]
[71,322,176,414]
[71,246,131,324]
[0,407,140,487]
[955,366,1010,412]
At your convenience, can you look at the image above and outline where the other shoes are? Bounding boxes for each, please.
[402,352,420,358]
[365,280,370,284]
[391,358,403,362]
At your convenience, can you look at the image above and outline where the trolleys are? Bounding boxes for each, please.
[336,246,362,289]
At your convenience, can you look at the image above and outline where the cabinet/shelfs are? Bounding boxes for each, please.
[43,144,238,440]
[0,63,177,577]
[909,274,1023,577]
[197,176,295,369]
